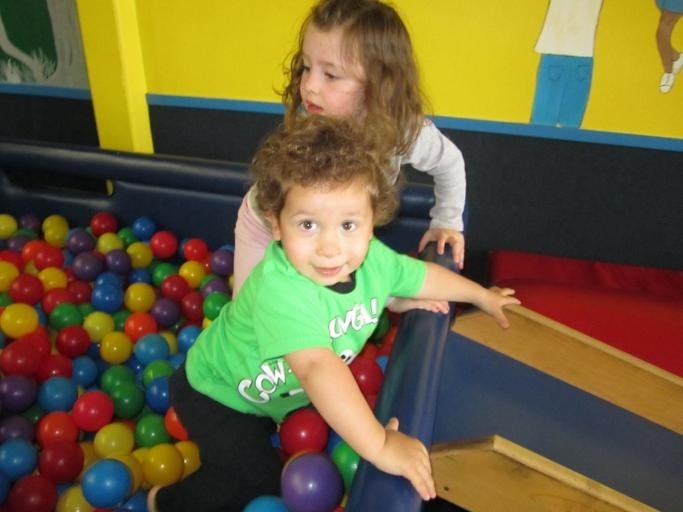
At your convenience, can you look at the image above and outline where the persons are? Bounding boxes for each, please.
[143,113,523,512]
[231,0,470,314]
